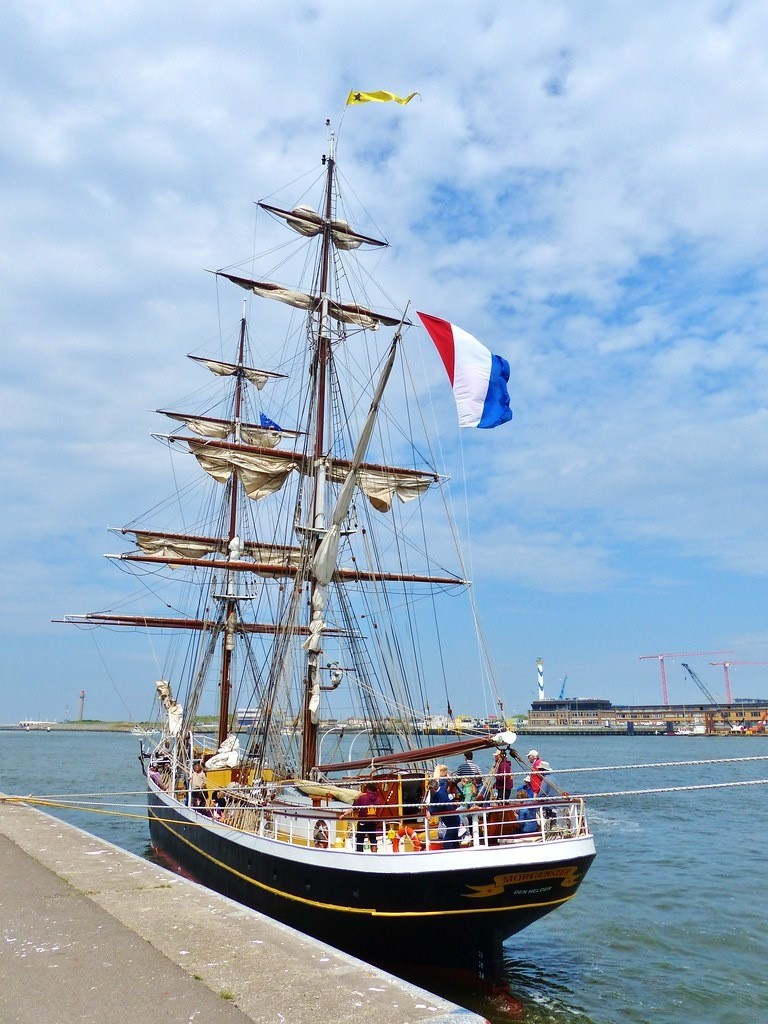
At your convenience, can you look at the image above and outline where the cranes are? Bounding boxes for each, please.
[710,658,767,708]
[637,649,730,707]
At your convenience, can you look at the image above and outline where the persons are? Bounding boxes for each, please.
[353,749,561,848]
[190,764,208,812]
[159,740,172,755]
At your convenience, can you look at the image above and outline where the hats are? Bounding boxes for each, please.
[536,761,553,771]
[524,775,531,782]
[493,749,501,755]
[526,750,538,757]
[365,783,377,791]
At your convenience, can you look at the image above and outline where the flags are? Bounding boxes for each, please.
[416,310,514,429]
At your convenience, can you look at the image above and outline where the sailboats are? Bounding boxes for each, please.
[133,113,603,954]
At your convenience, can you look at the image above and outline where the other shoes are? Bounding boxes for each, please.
[461,835,472,845]
[457,827,467,837]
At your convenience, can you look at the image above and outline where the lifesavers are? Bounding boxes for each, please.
[313,819,330,849]
[392,825,419,853]
